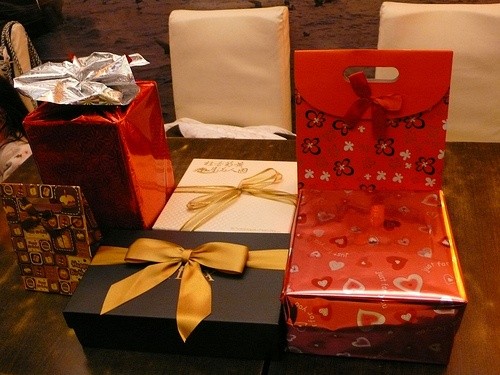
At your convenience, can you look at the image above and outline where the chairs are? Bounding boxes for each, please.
[378,1,500,142]
[165,6,293,136]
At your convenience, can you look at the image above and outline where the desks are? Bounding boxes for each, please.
[1,150,499,375]
